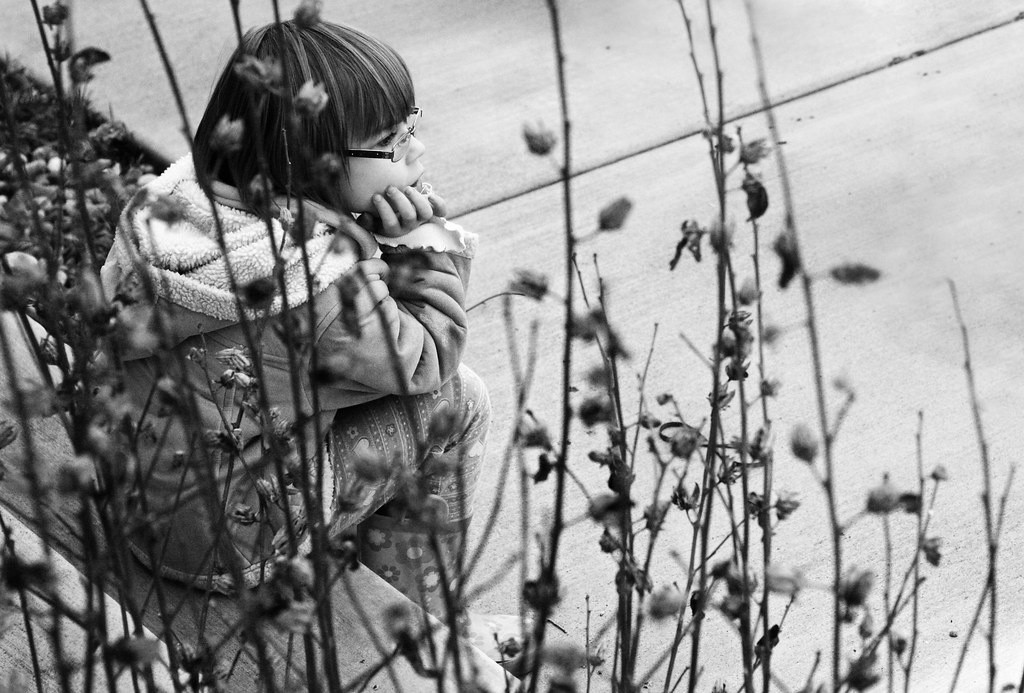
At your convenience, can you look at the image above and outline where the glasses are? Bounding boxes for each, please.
[333,106,424,163]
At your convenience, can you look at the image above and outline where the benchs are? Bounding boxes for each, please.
[0,311,553,693]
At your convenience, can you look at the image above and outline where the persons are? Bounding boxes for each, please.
[90,13,489,639]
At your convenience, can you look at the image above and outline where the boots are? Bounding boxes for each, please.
[357,495,545,676]
